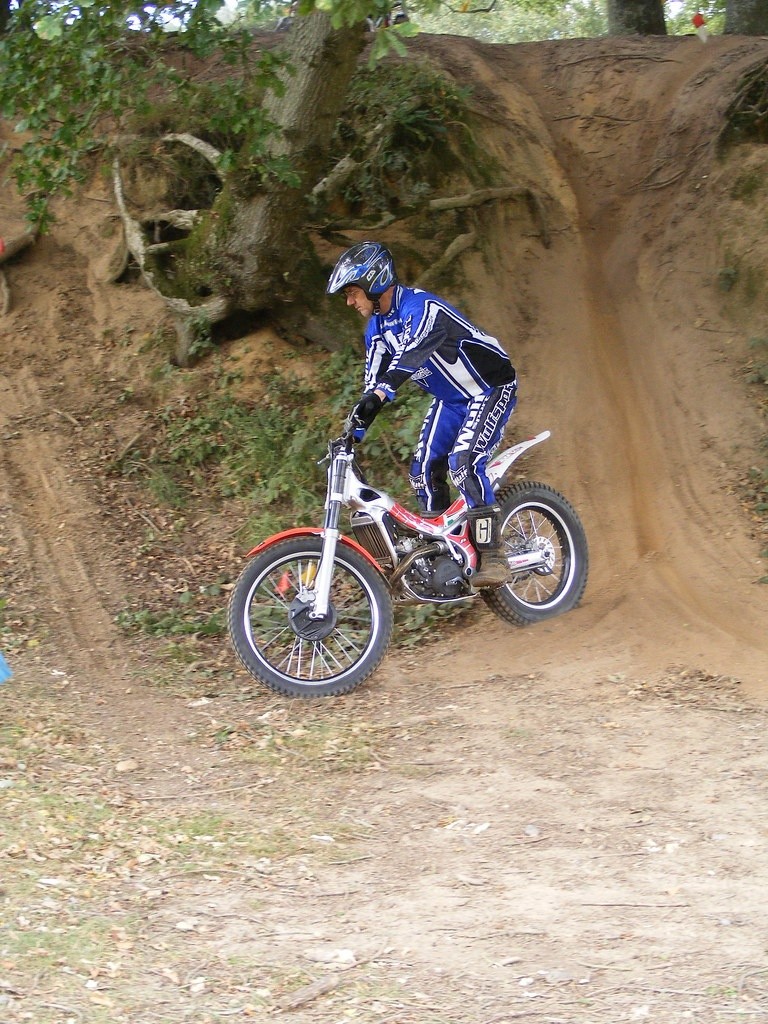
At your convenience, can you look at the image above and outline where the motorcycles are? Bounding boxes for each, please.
[226,400,590,699]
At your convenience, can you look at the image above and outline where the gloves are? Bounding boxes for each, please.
[330,432,358,451]
[344,393,382,428]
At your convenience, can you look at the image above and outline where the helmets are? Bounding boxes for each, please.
[325,241,398,301]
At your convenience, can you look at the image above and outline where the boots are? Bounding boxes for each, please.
[465,504,513,588]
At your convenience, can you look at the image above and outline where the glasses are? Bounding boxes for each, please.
[340,289,364,298]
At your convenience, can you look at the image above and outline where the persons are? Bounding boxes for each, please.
[326,242,519,586]
